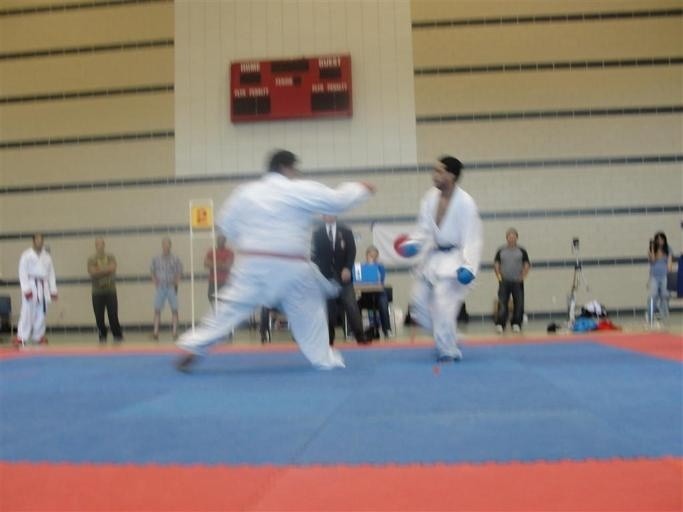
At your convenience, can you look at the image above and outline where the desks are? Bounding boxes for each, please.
[354,287,384,340]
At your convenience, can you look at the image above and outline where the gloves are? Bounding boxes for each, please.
[395,235,423,258]
[457,267,475,285]
[51,295,59,300]
[26,293,33,300]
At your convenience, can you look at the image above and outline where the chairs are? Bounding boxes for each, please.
[386,286,397,337]
[1,297,14,339]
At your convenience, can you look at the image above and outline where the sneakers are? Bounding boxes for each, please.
[14,339,24,345]
[386,330,394,339]
[495,324,503,335]
[512,324,521,334]
[39,338,48,344]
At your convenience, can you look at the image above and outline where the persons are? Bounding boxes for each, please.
[15,234,59,347]
[353,246,394,339]
[647,231,673,330]
[87,237,124,343]
[150,237,183,339]
[408,155,482,362]
[492,228,531,332]
[177,150,375,366]
[312,212,369,345]
[204,235,234,304]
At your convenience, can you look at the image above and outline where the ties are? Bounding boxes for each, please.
[329,224,334,274]
